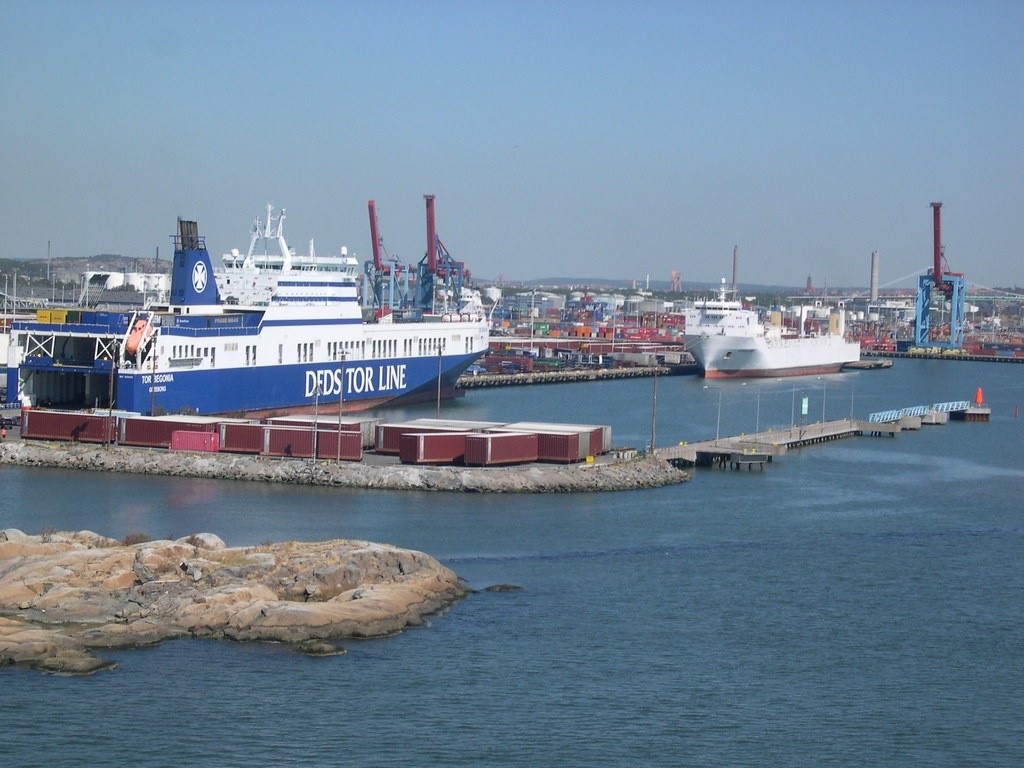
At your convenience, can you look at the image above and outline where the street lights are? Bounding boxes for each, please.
[740,383,760,441]
[775,378,796,438]
[701,386,722,447]
[816,376,827,431]
[839,374,855,428]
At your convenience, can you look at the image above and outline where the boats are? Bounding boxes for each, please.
[680,309,862,380]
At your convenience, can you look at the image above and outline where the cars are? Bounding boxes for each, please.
[467,365,486,373]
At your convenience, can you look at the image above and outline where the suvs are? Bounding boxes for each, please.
[0,417,14,431]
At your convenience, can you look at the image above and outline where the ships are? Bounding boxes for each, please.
[5,195,501,417]
